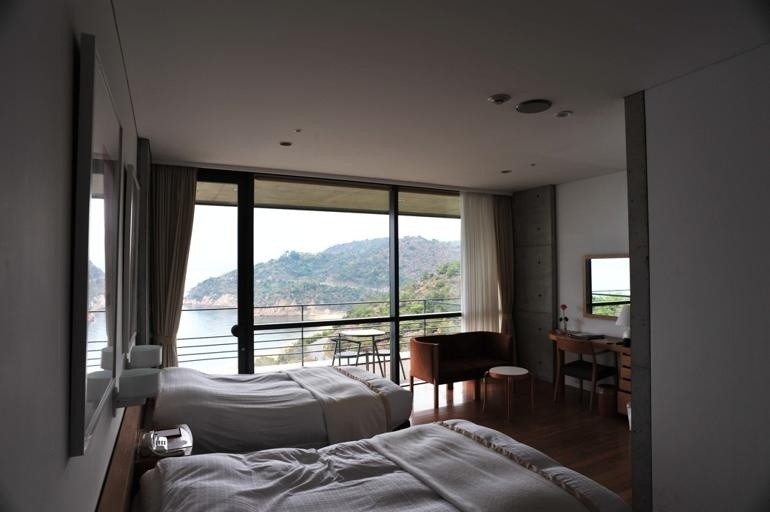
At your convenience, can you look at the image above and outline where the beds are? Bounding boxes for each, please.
[145,365,413,453]
[134,419,622,511]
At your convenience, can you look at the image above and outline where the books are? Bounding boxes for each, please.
[568,331,605,340]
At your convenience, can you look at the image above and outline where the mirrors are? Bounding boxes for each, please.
[580,254,631,321]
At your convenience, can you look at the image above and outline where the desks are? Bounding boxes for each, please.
[335,329,386,374]
[550,330,632,416]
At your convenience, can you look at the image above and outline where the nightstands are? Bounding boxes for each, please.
[136,423,194,475]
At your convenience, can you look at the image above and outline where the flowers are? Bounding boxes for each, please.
[558,304,568,331]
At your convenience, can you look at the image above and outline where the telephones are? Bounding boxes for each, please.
[140,430,169,457]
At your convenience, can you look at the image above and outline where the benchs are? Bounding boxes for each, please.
[409,330,514,410]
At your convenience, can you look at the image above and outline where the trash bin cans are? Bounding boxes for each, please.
[596,383,618,417]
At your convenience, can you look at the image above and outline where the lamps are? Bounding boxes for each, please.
[112,344,164,418]
[615,304,631,339]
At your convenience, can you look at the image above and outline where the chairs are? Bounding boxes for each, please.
[553,335,618,410]
[330,334,406,381]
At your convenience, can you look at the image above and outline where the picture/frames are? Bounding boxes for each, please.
[69,29,141,455]
